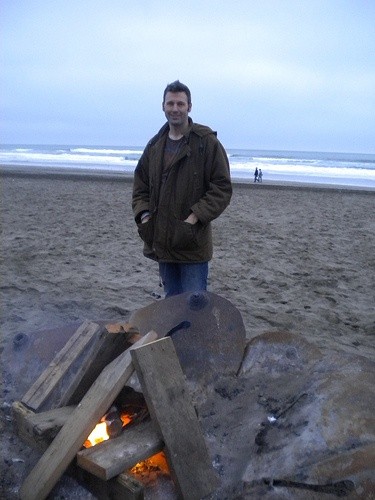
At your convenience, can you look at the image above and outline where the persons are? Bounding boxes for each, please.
[253,167,259,182]
[130,79,233,300]
[257,169,263,182]
